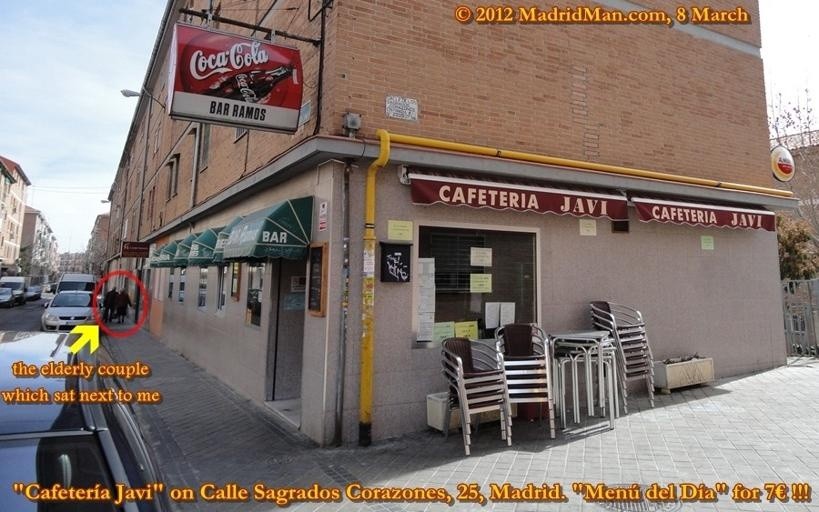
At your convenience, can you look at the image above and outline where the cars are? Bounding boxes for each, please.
[0,329,167,510]
[1,271,105,334]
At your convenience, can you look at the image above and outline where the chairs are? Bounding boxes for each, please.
[440,300,656,454]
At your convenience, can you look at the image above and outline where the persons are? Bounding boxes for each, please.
[103,286,132,323]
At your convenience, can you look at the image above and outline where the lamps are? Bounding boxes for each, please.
[120,88,166,112]
